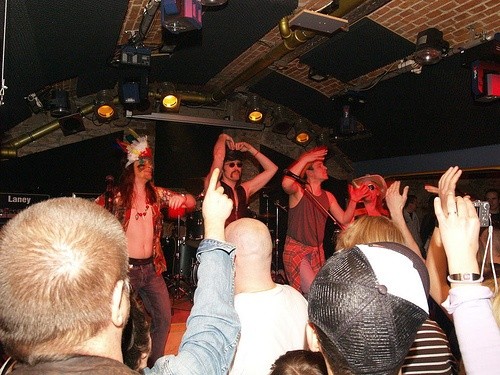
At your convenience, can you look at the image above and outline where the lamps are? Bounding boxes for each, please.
[48,91,314,148]
[413,27,449,66]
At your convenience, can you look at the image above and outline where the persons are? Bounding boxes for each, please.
[270,350,328,375]
[351,174,392,220]
[121,167,241,375]
[306,242,430,375]
[282,147,371,295]
[402,193,427,261]
[204,134,278,228]
[476,227,500,328]
[434,191,499,375]
[0,198,140,375]
[225,218,309,375]
[386,180,451,308]
[336,216,459,375]
[94,148,197,369]
[420,194,439,258]
[484,189,500,230]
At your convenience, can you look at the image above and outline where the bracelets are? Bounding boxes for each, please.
[254,150,260,156]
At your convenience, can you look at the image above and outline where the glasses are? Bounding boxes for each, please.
[368,185,379,190]
[224,163,243,167]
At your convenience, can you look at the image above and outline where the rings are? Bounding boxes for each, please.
[448,211,456,214]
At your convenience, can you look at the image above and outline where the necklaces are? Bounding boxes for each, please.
[132,199,149,221]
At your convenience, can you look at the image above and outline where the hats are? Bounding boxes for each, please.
[224,150,242,162]
[308,242,430,373]
[352,175,387,200]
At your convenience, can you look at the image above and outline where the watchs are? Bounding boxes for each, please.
[181,192,187,205]
[449,273,481,281]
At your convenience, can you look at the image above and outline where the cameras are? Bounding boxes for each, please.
[472,199,492,228]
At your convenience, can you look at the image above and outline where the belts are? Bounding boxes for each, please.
[129,256,152,263]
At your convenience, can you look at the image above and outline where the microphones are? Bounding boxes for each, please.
[351,179,360,188]
[273,199,288,213]
[283,168,308,184]
[104,174,114,198]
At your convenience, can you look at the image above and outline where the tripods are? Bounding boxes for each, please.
[167,215,193,304]
